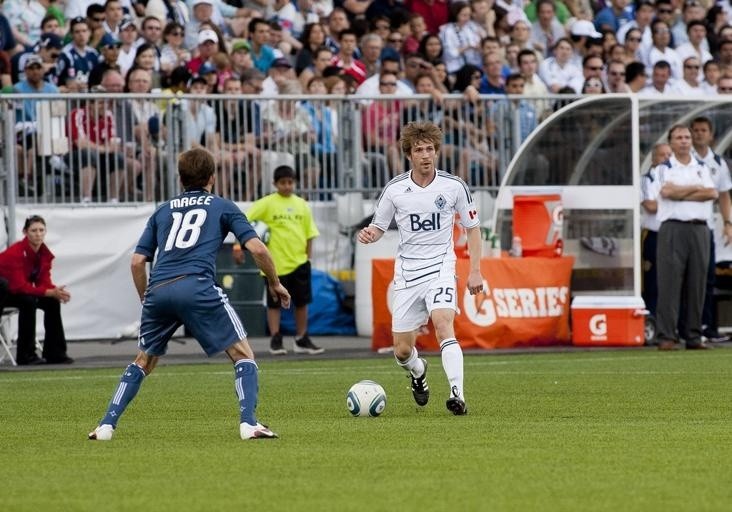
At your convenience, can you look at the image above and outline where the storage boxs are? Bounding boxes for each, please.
[570,294,651,347]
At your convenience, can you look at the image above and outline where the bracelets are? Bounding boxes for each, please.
[725,221,732,228]
[233,245,242,250]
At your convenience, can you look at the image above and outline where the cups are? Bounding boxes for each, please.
[480,223,501,258]
[510,236,523,257]
[126,141,135,157]
[110,137,121,152]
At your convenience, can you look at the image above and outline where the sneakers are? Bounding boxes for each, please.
[239,421,279,441]
[445,384,468,416]
[410,357,430,407]
[87,423,114,442]
[268,331,288,356]
[292,330,325,356]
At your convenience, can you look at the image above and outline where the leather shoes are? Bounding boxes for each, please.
[658,340,681,351]
[686,343,709,350]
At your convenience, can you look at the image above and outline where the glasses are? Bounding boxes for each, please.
[473,76,483,79]
[379,36,403,88]
[585,2,699,88]
[509,83,524,88]
[23,16,185,71]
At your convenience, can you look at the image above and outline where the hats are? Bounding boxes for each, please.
[185,29,293,93]
[569,19,603,40]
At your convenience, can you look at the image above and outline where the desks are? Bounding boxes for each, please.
[371,255,576,355]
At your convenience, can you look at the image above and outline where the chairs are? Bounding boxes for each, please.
[0,308,42,367]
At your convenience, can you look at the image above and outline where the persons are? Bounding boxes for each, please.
[0,214,75,365]
[232,163,325,355]
[655,125,720,350]
[680,115,732,344]
[1,0,731,203]
[88,149,292,442]
[640,143,673,343]
[358,121,483,416]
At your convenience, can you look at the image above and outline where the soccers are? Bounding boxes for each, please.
[347,379,386,417]
